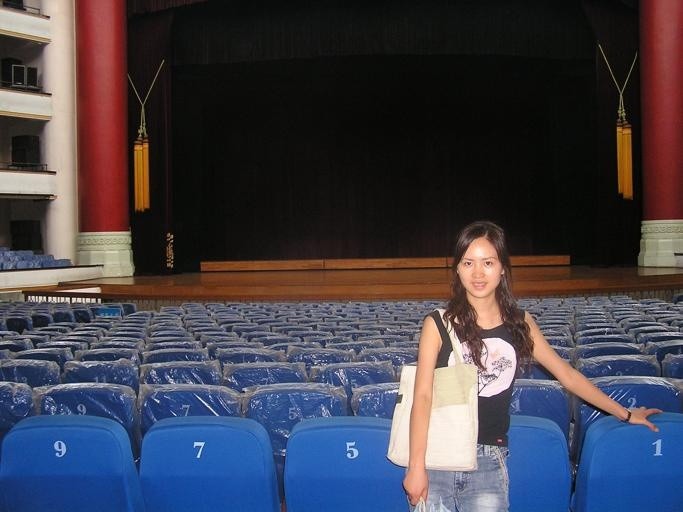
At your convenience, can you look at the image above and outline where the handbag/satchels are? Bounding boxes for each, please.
[386,308,480,472]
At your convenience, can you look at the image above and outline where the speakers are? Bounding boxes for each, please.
[3,1,24,11]
[9,220,41,252]
[11,65,24,85]
[27,67,37,87]
[11,135,41,165]
[1,56,21,84]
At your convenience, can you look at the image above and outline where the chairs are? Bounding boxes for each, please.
[0,247,73,270]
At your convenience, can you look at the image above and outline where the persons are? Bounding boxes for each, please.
[400,220,664,512]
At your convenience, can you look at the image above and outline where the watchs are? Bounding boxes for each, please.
[621,406,633,422]
